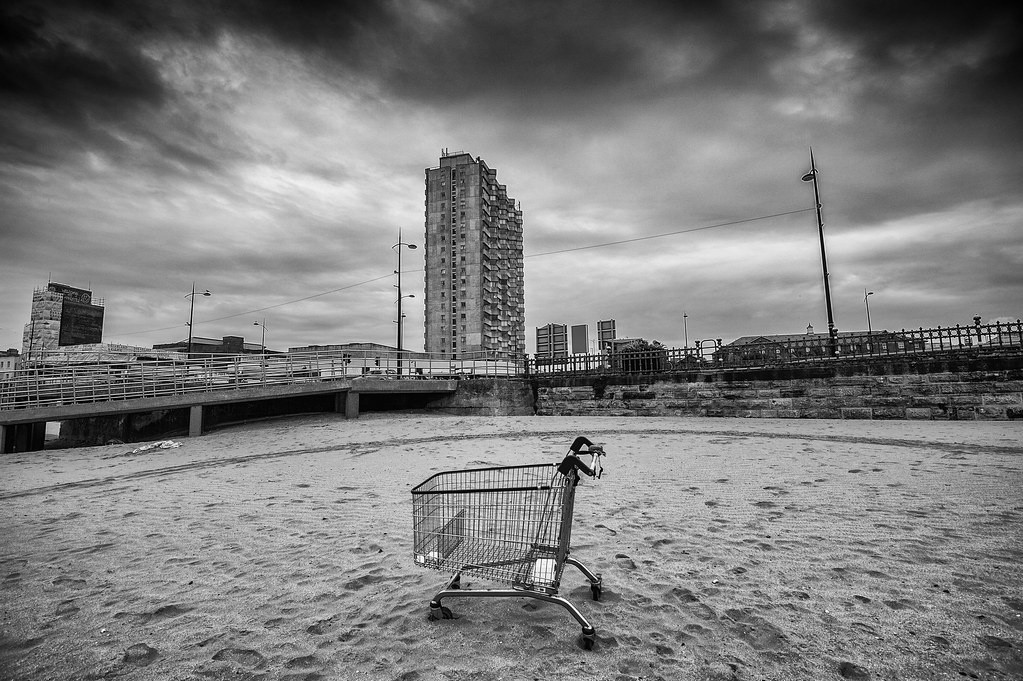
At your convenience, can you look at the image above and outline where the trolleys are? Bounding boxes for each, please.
[410,436,606,652]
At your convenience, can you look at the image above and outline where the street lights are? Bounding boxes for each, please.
[254,316,268,353]
[863,288,874,353]
[802,145,839,357]
[183,280,211,374]
[684,312,688,348]
[391,224,417,379]
[25,317,49,361]
